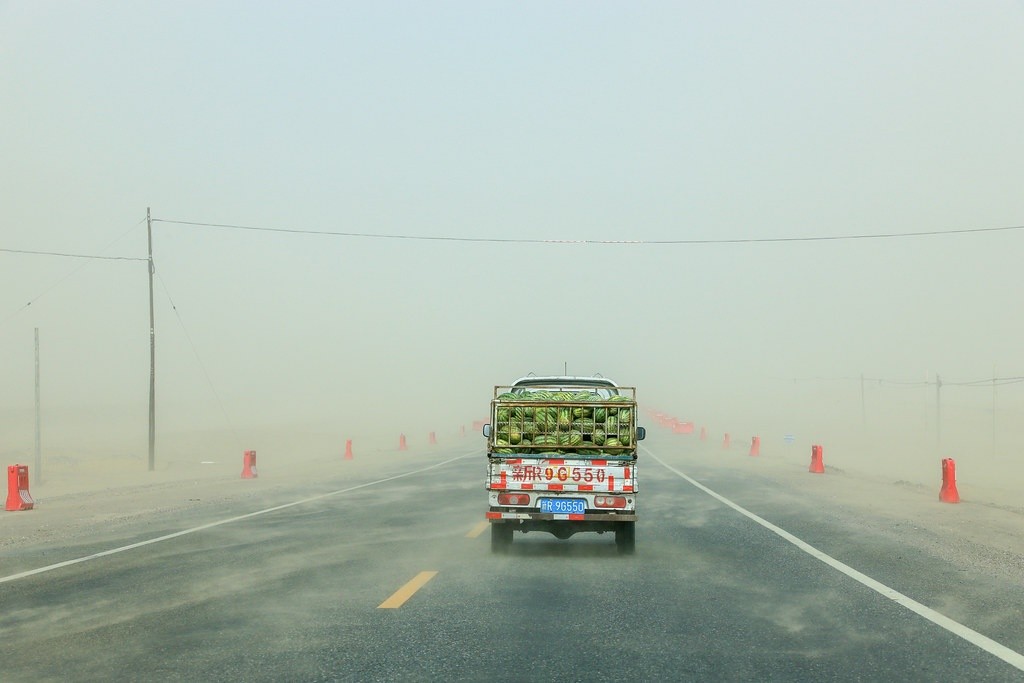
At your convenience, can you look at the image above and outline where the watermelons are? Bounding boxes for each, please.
[493,392,634,456]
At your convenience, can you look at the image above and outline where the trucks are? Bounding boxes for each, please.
[483,372,647,553]
